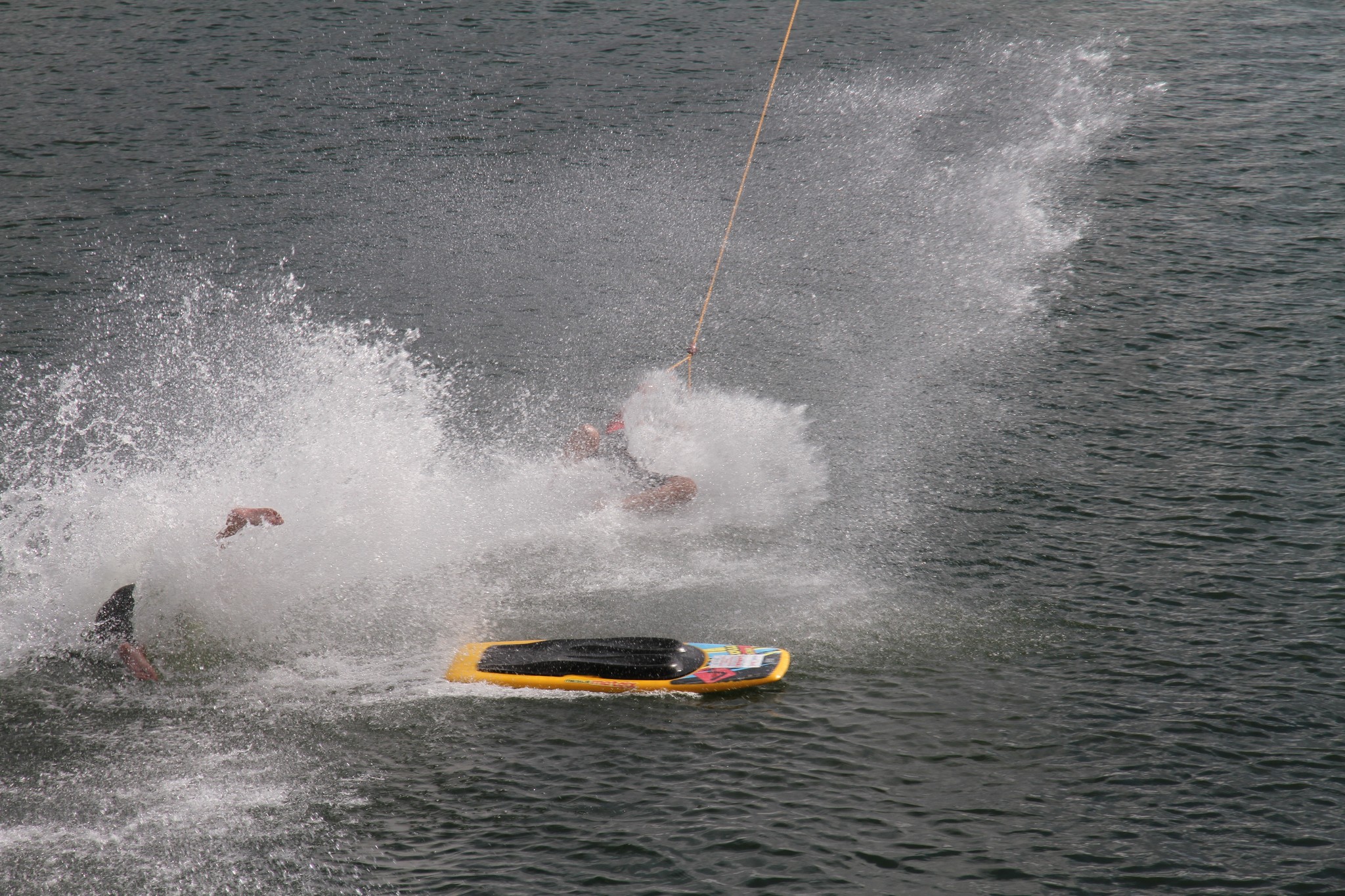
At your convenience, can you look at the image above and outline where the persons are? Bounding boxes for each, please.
[566,422,698,515]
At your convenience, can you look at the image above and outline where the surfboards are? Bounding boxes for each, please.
[444,637,791,694]
